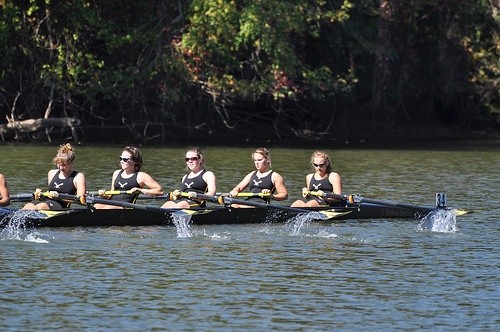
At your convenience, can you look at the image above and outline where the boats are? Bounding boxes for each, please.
[0,191,453,227]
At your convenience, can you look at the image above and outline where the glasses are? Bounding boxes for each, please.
[314,163,324,167]
[184,157,198,162]
[119,155,130,163]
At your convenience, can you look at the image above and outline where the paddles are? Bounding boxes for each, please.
[307,192,473,216]
[53,192,196,215]
[178,192,352,220]
[85,190,133,196]
[215,192,270,196]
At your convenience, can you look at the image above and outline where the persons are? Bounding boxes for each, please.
[93,146,161,209]
[22,142,86,210]
[290,151,342,207]
[228,148,288,208]
[0,173,10,207]
[160,145,216,208]
